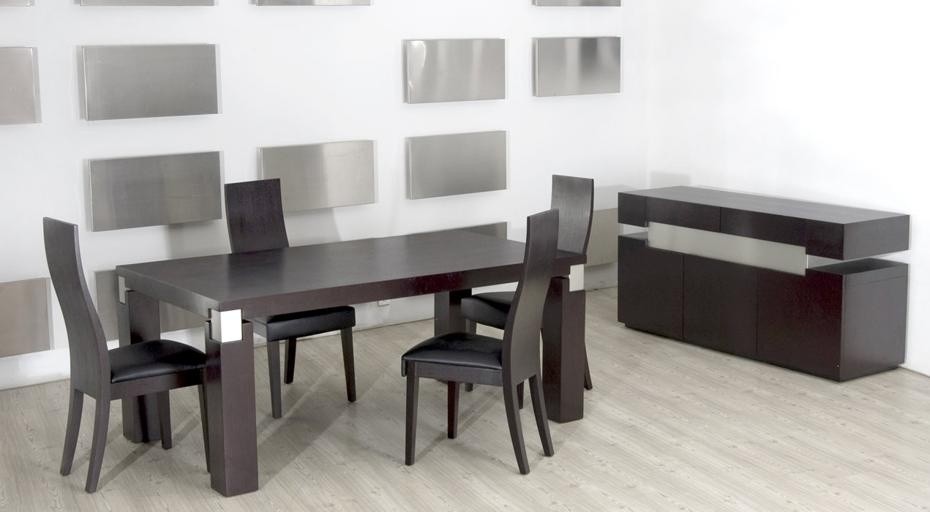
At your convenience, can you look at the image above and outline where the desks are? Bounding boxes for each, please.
[113,225,587,496]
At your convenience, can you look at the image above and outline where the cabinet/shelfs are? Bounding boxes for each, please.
[617,185,910,381]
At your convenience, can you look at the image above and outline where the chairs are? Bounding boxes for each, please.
[400,209,554,473]
[43,217,210,493]
[225,178,357,418]
[460,174,595,408]
